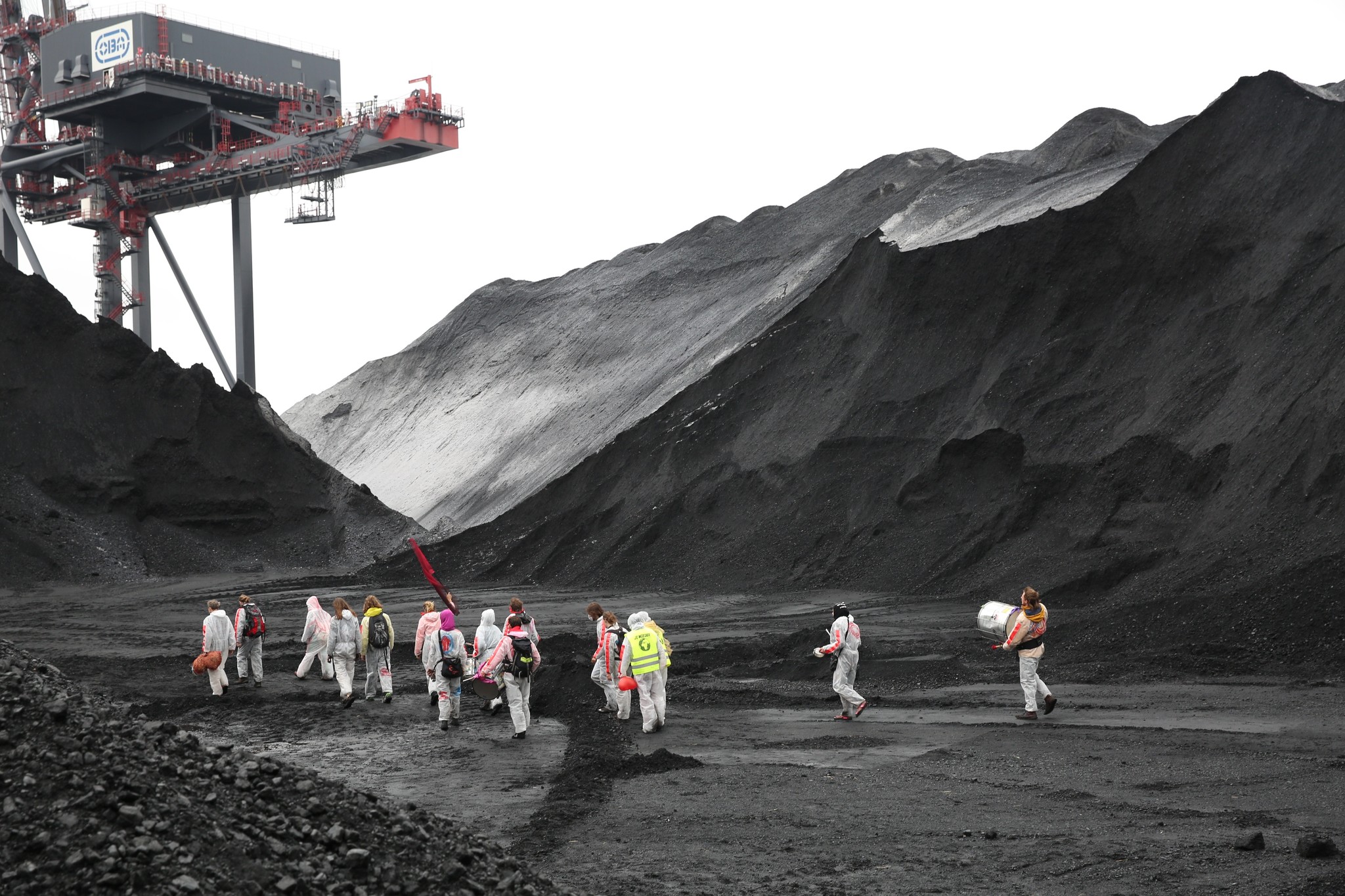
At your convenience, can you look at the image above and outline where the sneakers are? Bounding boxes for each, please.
[1043,695,1057,715]
[1015,711,1037,719]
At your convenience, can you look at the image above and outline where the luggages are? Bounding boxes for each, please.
[480,700,491,710]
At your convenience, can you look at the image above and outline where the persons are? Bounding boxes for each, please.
[294,596,335,680]
[586,603,673,734]
[326,597,363,708]
[414,596,541,738]
[359,595,394,704]
[1001,587,1057,720]
[233,594,265,687]
[202,599,236,697]
[819,601,869,721]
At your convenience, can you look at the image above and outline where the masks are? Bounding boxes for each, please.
[588,614,594,621]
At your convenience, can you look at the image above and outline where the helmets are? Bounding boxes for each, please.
[618,676,638,691]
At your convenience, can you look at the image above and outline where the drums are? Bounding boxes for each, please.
[463,658,476,678]
[977,601,1024,643]
[813,647,825,658]
[472,661,506,700]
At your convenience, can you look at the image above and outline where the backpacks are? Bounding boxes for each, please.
[368,612,389,647]
[604,626,627,656]
[237,604,265,638]
[502,635,533,677]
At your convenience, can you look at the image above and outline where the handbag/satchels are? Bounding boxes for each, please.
[442,658,463,678]
[829,655,838,671]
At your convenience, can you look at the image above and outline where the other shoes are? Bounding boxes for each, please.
[295,671,304,680]
[254,682,261,687]
[491,704,502,716]
[597,707,614,713]
[512,731,526,739]
[441,720,448,730]
[430,691,438,706]
[321,677,333,681]
[234,677,248,684]
[613,715,628,722]
[450,717,459,725]
[342,692,392,709]
[222,685,228,695]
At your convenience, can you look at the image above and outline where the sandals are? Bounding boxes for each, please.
[834,715,852,720]
[856,701,868,717]
